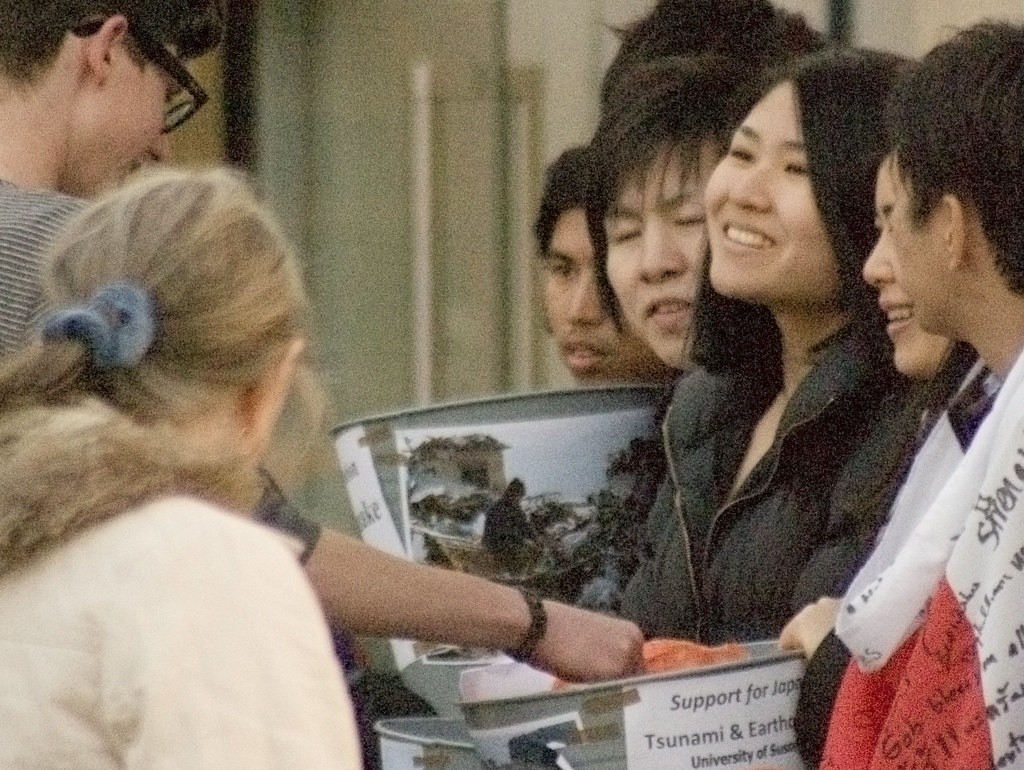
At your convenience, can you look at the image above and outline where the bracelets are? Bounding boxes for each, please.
[508,585,547,663]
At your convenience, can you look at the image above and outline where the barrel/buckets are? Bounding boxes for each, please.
[373,716,479,769]
[326,384,677,714]
[455,641,806,769]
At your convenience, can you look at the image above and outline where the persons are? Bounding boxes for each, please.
[0,170,364,770]
[483,479,544,574]
[535,0,1024,770]
[0,0,642,687]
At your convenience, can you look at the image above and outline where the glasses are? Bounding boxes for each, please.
[69,16,209,136]
